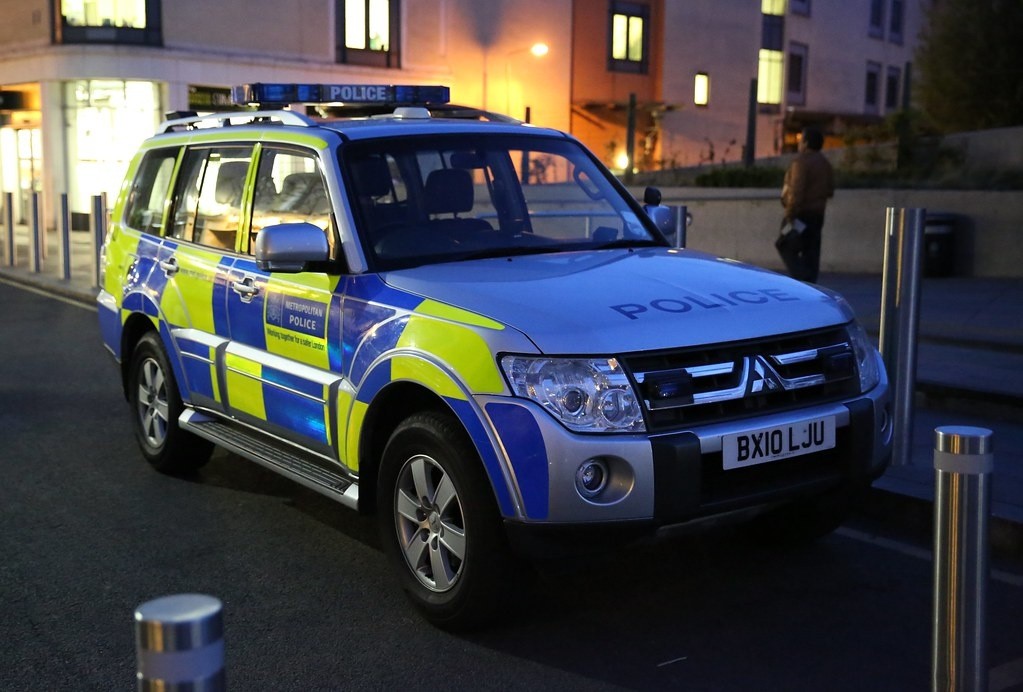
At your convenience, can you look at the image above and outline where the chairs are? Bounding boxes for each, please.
[422,167,497,243]
[200,162,278,256]
[271,172,332,229]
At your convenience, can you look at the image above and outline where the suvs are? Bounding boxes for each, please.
[97,82,900,627]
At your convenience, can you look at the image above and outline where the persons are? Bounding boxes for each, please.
[773,125,834,281]
